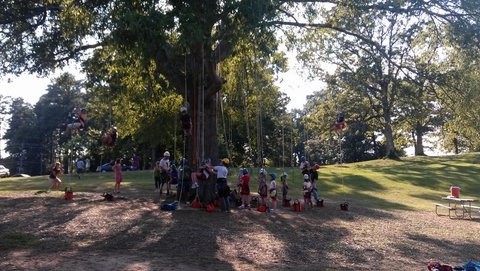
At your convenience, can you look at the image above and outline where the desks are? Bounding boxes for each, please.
[442,195,474,219]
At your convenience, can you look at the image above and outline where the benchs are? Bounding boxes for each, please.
[461,205,480,217]
[435,204,458,220]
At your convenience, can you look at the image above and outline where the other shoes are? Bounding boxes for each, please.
[238,204,250,208]
[222,208,231,213]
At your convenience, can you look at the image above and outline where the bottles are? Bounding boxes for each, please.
[294,199,300,212]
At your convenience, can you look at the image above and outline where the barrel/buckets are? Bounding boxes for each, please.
[451,187,460,198]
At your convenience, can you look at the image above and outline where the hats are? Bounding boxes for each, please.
[315,164,320,170]
[303,174,310,179]
[270,173,277,180]
[164,151,170,157]
[259,168,267,175]
[301,156,305,161]
[281,172,288,179]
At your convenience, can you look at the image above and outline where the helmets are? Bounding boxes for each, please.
[221,157,230,164]
[239,168,248,174]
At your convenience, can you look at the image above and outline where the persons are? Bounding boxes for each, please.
[179,106,193,135]
[155,151,322,212]
[334,111,346,139]
[50,108,140,192]
